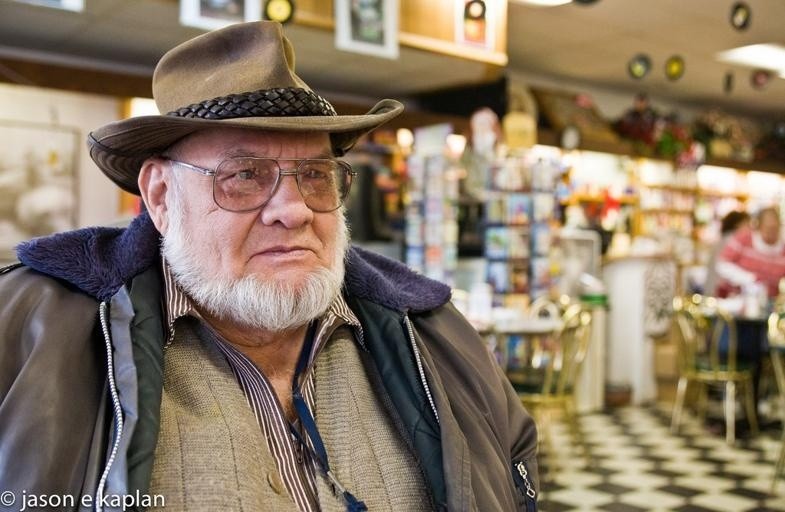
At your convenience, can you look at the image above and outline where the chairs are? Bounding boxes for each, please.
[446,284,595,479]
[669,275,785,493]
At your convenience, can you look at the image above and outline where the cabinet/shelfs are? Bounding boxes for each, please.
[556,147,785,253]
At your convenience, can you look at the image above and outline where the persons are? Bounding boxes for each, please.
[0,20,542,510]
[703,211,784,436]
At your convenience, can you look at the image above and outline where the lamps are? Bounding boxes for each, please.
[395,128,416,159]
[440,133,467,161]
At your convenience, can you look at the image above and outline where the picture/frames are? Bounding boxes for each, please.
[179,0,262,33]
[19,0,84,13]
[333,0,400,61]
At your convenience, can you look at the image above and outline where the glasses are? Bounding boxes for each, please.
[153,155,358,212]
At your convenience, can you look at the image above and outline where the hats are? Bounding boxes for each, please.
[85,20,405,197]
